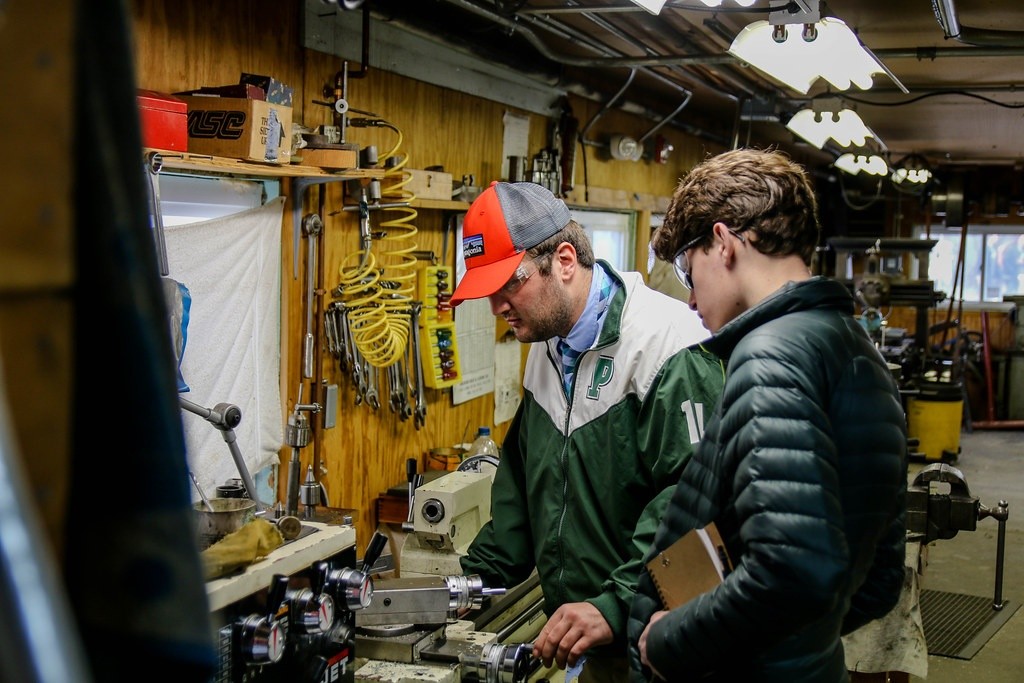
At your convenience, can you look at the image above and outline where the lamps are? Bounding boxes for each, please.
[629,0,933,185]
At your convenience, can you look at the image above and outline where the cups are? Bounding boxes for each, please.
[194,498,257,550]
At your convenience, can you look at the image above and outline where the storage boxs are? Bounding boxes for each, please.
[176,95,293,164]
[296,149,356,168]
[381,168,453,200]
[136,88,189,151]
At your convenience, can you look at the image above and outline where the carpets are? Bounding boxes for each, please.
[920,589,1023,660]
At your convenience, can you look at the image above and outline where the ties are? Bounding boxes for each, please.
[559,343,581,403]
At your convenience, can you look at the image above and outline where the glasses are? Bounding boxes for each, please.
[500,248,557,294]
[673,228,745,291]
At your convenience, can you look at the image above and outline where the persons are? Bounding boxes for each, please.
[626,145,906,683]
[448,180,724,683]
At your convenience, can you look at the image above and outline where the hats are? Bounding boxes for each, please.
[449,181,572,307]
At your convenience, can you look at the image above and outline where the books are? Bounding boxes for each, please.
[645,522,734,611]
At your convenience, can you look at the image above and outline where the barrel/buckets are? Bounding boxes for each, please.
[901,379,968,459]
